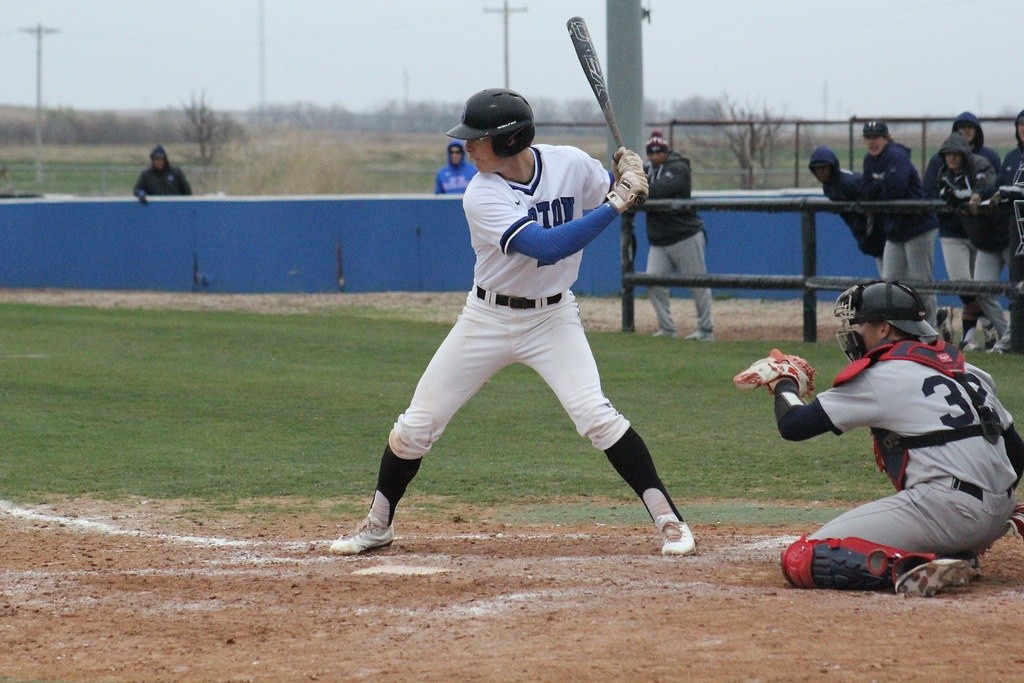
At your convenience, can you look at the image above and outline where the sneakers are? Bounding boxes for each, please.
[935,306,1011,355]
[329,514,394,556]
[658,520,697,556]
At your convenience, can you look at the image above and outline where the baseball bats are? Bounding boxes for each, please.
[566,16,648,206]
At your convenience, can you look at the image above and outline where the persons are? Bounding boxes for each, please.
[808,109,1024,356]
[330,87,696,555]
[434,139,478,194]
[133,145,209,286]
[734,283,1024,598]
[642,128,714,342]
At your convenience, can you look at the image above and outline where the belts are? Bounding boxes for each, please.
[476,285,562,310]
[953,477,1014,500]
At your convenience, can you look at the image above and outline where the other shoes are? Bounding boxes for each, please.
[895,552,982,597]
[652,328,715,342]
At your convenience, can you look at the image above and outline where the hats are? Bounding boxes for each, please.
[863,122,888,137]
[1016,116,1024,124]
[810,162,831,167]
[152,153,166,160]
[956,121,976,130]
[646,129,668,153]
[941,148,962,154]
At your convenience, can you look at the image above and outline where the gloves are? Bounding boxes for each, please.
[140,189,147,204]
[1006,503,1024,539]
[606,148,649,215]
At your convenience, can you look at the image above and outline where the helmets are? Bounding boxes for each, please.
[847,282,939,337]
[446,88,536,157]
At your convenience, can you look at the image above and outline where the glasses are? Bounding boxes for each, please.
[646,147,666,154]
[449,150,462,154]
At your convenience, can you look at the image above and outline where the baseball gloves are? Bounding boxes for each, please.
[733,348,817,398]
